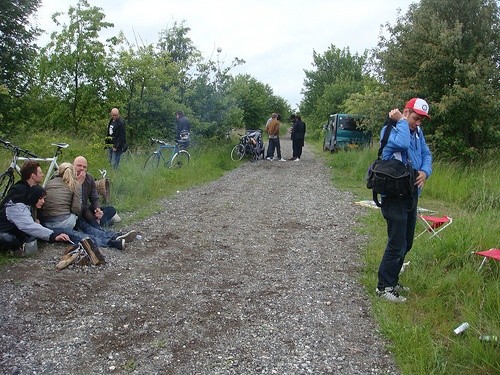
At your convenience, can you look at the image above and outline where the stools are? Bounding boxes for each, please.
[471,248,500,276]
[414,211,452,247]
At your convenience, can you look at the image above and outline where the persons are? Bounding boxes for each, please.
[375,98,433,302]
[106,108,127,166]
[290,114,306,160]
[0,157,138,250]
[173,109,191,154]
[353,113,372,150]
[265,113,288,162]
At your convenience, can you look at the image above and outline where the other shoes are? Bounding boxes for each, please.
[271,156,274,161]
[278,157,286,161]
[112,229,137,242]
[375,287,406,303]
[392,283,410,292]
[266,156,270,159]
[289,156,295,160]
[109,238,125,249]
[294,157,300,161]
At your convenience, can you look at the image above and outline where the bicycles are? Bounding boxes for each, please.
[143,136,191,173]
[0,139,70,201]
[230,132,261,163]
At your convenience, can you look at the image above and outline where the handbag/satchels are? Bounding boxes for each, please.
[366,112,415,198]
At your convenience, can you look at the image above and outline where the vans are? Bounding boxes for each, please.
[322,113,373,154]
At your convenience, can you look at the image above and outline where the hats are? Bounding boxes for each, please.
[404,97,431,122]
